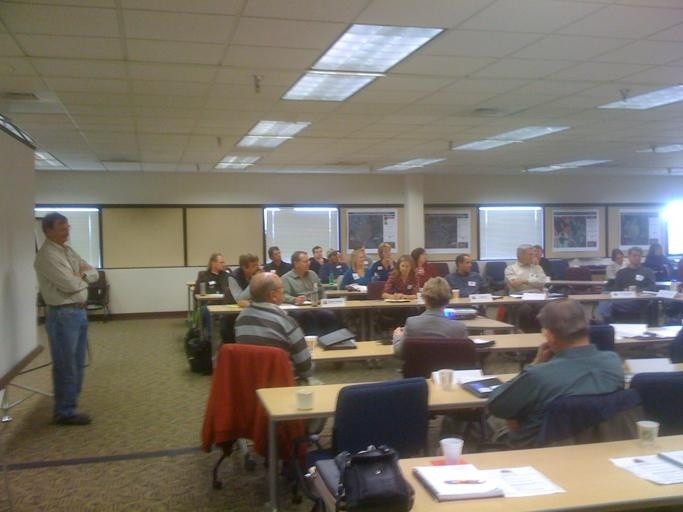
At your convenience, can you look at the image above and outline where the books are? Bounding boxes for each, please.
[617,355,673,386]
[387,297,411,302]
[413,462,503,504]
[319,328,359,351]
[464,379,505,402]
[654,449,683,471]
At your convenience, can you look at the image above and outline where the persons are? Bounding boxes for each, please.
[486,289,629,447]
[603,248,627,282]
[231,272,316,385]
[611,247,658,291]
[443,253,489,298]
[33,210,99,428]
[390,277,471,381]
[278,250,327,306]
[533,245,559,283]
[411,244,440,292]
[220,255,264,304]
[642,241,672,275]
[189,251,232,302]
[381,255,420,300]
[371,241,399,283]
[338,248,374,292]
[262,245,291,279]
[503,243,548,296]
[307,245,329,271]
[317,250,348,284]
[356,244,373,267]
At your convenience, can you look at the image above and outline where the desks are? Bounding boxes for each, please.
[303,333,675,362]
[256,358,682,512]
[185,281,197,320]
[205,292,683,374]
[194,281,670,343]
[307,434,683,511]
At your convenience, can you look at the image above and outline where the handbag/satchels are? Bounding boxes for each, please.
[335,444,415,512]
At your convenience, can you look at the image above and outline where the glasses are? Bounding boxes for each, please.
[272,287,284,293]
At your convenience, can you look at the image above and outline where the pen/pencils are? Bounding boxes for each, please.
[444,480,485,485]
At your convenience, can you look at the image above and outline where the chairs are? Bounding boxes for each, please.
[220,313,238,343]
[86,271,112,324]
[631,372,683,436]
[479,387,644,452]
[292,377,429,512]
[589,325,615,351]
[428,262,450,277]
[213,344,327,505]
[472,262,478,273]
[565,268,596,292]
[548,260,569,280]
[36,290,46,326]
[403,338,493,378]
[291,308,349,335]
[486,262,507,294]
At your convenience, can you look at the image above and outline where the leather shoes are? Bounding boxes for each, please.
[55,414,92,426]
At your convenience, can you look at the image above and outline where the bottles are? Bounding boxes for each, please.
[658,298,666,327]
[311,282,318,308]
[198,270,206,296]
[649,299,659,327]
[329,271,334,285]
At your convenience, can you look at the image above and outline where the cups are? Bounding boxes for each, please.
[634,419,660,449]
[438,369,454,390]
[438,437,464,465]
[451,289,460,300]
[294,387,314,409]
[303,336,318,353]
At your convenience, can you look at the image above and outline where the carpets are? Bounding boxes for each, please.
[0,318,402,511]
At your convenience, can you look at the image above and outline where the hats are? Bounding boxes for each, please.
[326,248,339,258]
